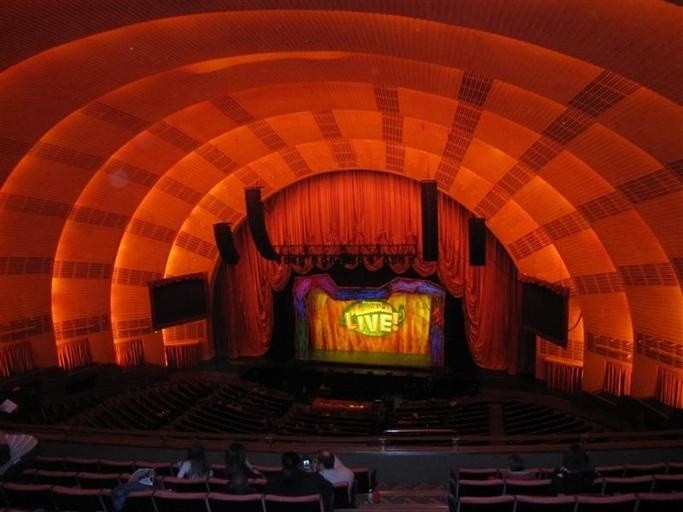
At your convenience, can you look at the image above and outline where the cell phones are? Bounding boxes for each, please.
[303,460,310,470]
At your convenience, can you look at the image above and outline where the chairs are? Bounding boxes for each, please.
[1,454,380,511]
[448,461,682,512]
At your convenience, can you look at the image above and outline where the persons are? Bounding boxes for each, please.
[550,444,606,496]
[176,442,215,478]
[223,442,266,477]
[217,475,257,493]
[311,448,355,503]
[263,451,320,496]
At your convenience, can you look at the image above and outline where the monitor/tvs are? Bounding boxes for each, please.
[518,274,570,350]
[146,272,211,331]
